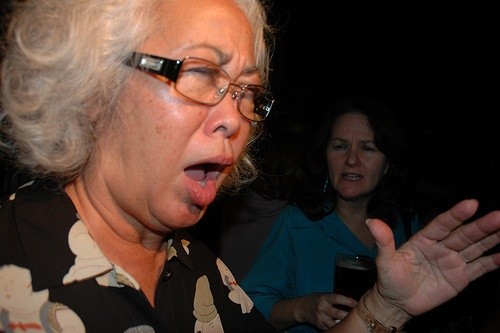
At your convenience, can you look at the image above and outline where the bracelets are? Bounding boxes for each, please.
[356,290,397,333]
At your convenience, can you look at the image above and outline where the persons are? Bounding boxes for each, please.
[0,0,499,333]
[236,106,396,330]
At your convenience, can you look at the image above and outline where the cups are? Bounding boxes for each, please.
[332,252,377,324]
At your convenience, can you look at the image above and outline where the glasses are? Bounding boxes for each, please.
[118,45,278,126]
